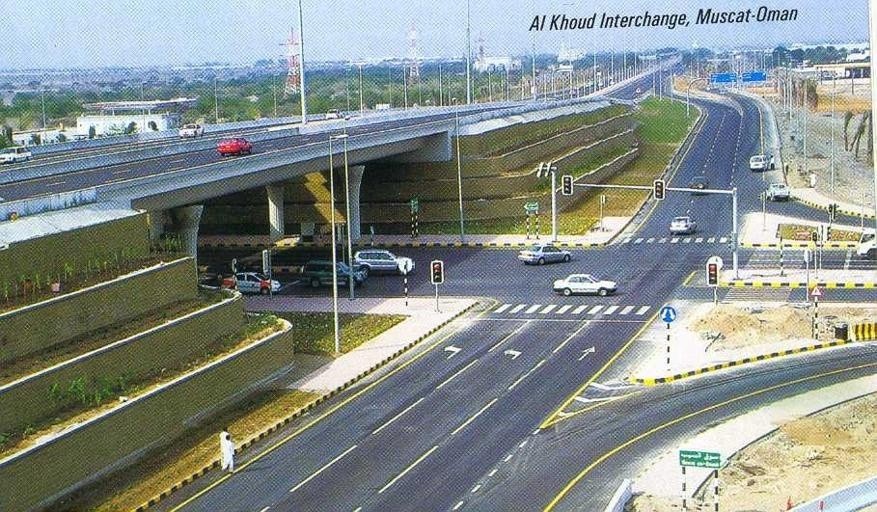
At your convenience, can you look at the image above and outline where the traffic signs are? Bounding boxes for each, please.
[524,201,539,211]
[410,195,418,214]
[742,72,767,82]
[711,73,736,82]
[679,450,720,469]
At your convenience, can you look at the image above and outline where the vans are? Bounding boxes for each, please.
[353,250,416,275]
[749,154,768,172]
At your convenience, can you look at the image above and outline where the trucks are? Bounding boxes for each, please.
[856,231,877,260]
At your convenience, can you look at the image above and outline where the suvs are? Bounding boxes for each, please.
[299,261,365,289]
[766,183,790,201]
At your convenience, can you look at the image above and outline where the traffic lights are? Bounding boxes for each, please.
[834,203,839,219]
[432,261,442,283]
[654,181,664,198]
[709,264,717,285]
[603,196,608,204]
[824,226,831,240]
[813,232,817,241]
[829,205,832,213]
[564,178,571,194]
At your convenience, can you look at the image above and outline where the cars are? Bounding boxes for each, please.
[669,217,698,235]
[689,176,708,190]
[326,108,344,119]
[553,274,617,297]
[218,137,253,156]
[569,80,604,95]
[518,245,572,265]
[0,146,32,164]
[234,273,281,294]
[179,124,205,139]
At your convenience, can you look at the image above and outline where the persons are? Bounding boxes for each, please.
[219,427,229,467]
[222,435,235,472]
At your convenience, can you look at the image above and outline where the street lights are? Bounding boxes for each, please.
[273,72,280,119]
[213,75,221,129]
[451,96,468,240]
[329,133,350,355]
[341,115,356,300]
[142,79,148,134]
